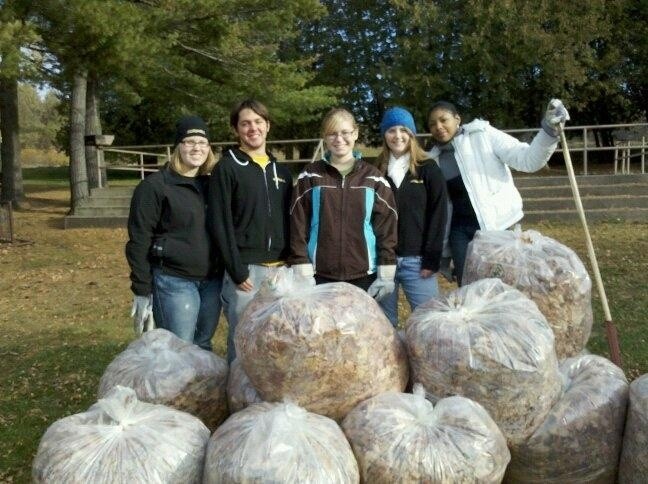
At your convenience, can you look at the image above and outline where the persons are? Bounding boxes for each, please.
[287,107,399,303]
[420,97,571,288]
[208,97,294,362]
[126,113,226,351]
[370,106,449,330]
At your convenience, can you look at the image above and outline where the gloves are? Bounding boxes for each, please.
[540,98,570,137]
[291,263,316,286]
[367,265,397,303]
[130,293,153,337]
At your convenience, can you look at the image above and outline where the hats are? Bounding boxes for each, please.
[174,116,209,150]
[380,107,417,136]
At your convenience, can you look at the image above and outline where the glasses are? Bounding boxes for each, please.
[326,129,355,140]
[180,140,208,147]
